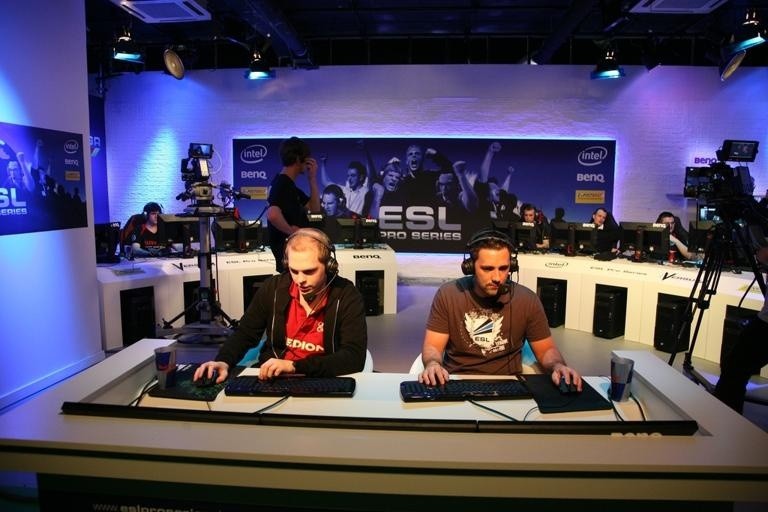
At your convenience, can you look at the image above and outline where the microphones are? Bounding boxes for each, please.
[307,274,337,302]
[499,273,512,295]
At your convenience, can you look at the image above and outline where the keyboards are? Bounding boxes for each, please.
[400,378,532,403]
[224,376,356,397]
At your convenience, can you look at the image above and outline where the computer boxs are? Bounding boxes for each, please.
[183,278,216,325]
[119,285,156,348]
[537,277,567,328]
[593,283,628,339]
[243,274,273,314]
[653,292,690,354]
[720,305,768,376]
[355,270,386,316]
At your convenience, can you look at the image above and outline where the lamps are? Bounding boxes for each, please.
[114,34,142,65]
[719,41,746,83]
[246,45,274,81]
[734,8,764,53]
[165,35,208,80]
[589,50,621,81]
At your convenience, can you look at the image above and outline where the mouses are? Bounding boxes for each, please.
[197,369,218,387]
[558,376,577,394]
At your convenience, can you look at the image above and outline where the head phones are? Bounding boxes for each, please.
[281,233,339,276]
[320,185,346,209]
[460,229,519,275]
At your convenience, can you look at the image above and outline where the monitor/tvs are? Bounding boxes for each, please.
[494,222,537,252]
[548,221,598,256]
[214,219,262,253]
[687,220,718,271]
[620,222,671,262]
[157,213,200,258]
[94,220,122,264]
[334,217,380,248]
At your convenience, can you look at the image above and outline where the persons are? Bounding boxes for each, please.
[319,138,520,222]
[417,228,583,395]
[268,136,321,273]
[657,213,695,259]
[517,203,551,249]
[592,208,609,252]
[714,248,768,413]
[550,207,566,223]
[130,203,166,251]
[0,137,86,227]
[193,229,367,385]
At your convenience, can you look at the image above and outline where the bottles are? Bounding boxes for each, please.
[669,250,676,263]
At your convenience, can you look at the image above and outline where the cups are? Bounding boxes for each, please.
[154,346,177,390]
[132,243,140,256]
[609,357,634,402]
[124,245,132,259]
